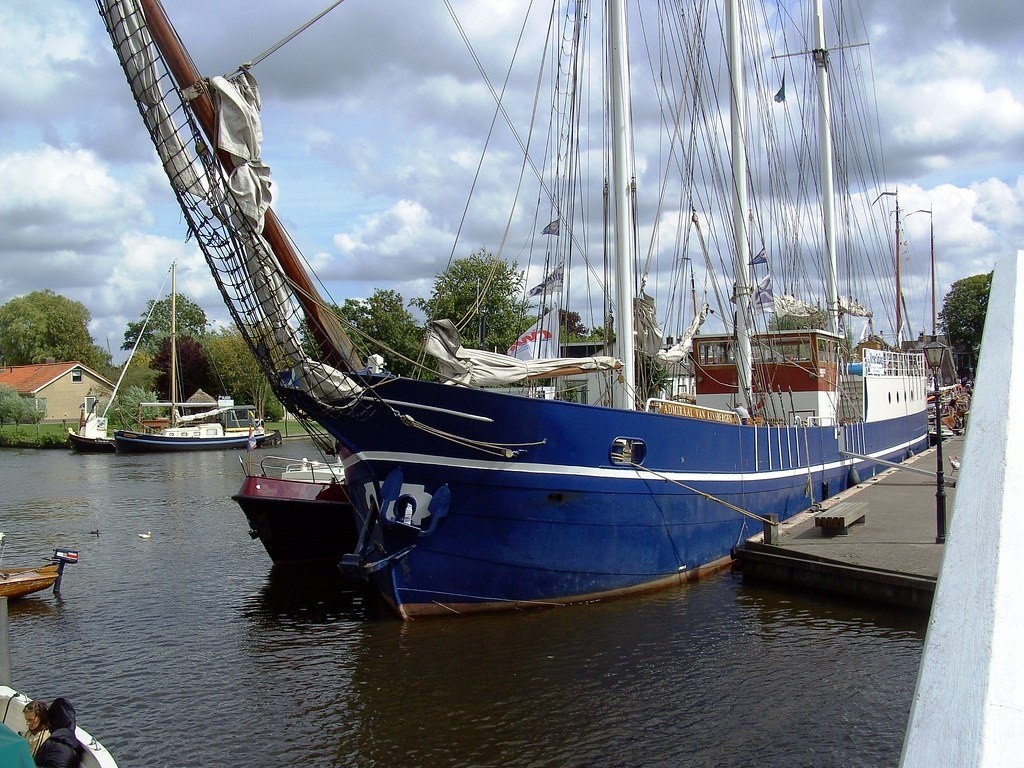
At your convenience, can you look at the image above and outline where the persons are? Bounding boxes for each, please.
[33,698,81,768]
[22,700,51,757]
[732,402,750,425]
[948,399,963,436]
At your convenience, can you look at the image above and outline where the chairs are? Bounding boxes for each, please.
[947,455,960,483]
[942,405,965,436]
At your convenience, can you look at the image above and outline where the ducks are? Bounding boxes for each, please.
[138,531,152,539]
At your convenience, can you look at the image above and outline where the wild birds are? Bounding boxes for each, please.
[89,529,99,534]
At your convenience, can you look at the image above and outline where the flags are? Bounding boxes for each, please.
[247,427,257,452]
[507,306,559,361]
[774,71,785,103]
[751,274,776,314]
[526,261,564,297]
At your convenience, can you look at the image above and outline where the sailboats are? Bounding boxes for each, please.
[67,258,283,454]
[108,0,934,624]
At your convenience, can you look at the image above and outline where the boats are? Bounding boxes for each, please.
[926,365,975,446]
[0,532,78,601]
[231,449,364,576]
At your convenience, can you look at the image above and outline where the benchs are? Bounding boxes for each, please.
[815,501,870,536]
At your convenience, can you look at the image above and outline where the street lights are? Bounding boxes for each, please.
[922,330,947,545]
